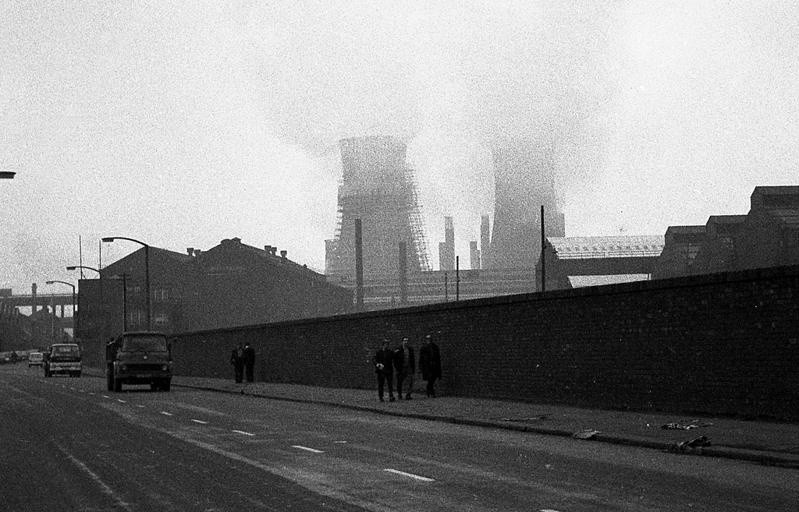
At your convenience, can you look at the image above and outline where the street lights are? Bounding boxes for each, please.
[66,265,104,336]
[0,171,16,179]
[46,280,76,343]
[102,236,152,334]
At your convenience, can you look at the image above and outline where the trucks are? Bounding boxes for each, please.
[41,342,81,379]
[28,351,43,368]
[103,328,172,393]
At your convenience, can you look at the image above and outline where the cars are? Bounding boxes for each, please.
[0,349,40,364]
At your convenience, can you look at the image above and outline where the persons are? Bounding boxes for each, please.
[418,335,442,398]
[243,342,256,383]
[10,349,19,363]
[392,336,414,400]
[372,339,395,401]
[230,343,244,383]
